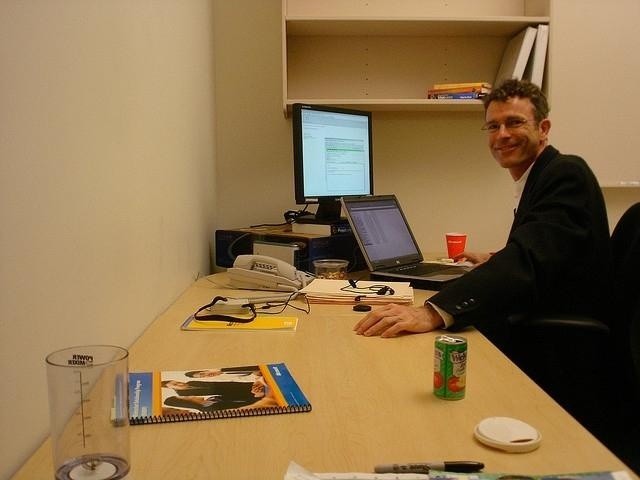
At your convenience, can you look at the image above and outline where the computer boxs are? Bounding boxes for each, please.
[215,223,366,277]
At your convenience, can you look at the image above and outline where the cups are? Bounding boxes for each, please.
[45,345,130,479]
[445,233,466,261]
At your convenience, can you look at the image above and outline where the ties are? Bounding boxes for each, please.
[513,206,518,221]
[221,371,255,375]
[185,386,195,390]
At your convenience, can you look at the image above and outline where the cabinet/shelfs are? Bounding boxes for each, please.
[281,0,554,120]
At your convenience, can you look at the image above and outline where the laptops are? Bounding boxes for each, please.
[340,194,475,286]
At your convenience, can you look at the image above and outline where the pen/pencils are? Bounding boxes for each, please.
[372,461,486,472]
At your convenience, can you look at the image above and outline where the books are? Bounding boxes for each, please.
[494,24,549,91]
[181,314,298,332]
[298,278,415,307]
[109,361,313,425]
[428,82,493,99]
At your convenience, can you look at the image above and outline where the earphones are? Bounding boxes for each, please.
[389,288,394,294]
[355,295,366,301]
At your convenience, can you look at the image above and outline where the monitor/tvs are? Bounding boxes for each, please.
[293,103,373,225]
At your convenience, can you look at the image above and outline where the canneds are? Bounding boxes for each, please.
[433,334,467,401]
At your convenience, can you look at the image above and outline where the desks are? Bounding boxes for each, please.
[0,264,639,480]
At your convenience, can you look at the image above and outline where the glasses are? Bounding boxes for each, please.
[480,119,539,134]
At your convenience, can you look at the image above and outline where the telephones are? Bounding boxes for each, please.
[227,254,316,292]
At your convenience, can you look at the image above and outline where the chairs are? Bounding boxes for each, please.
[485,199,639,341]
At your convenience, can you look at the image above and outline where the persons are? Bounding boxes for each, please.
[162,366,281,415]
[352,78,611,338]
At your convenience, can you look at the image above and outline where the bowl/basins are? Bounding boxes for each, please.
[312,259,349,280]
